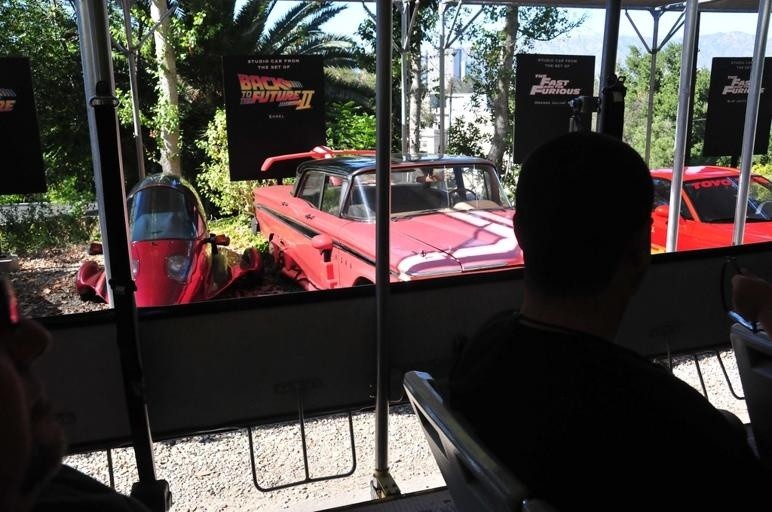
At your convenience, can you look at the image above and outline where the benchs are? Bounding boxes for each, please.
[654,185,756,216]
[316,184,447,215]
[730,323,771,462]
[401,370,554,510]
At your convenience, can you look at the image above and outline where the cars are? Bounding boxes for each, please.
[252,146,528,294]
[647,165,772,251]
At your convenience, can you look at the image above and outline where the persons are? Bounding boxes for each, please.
[442,127,772,511]
[0,266,161,511]
